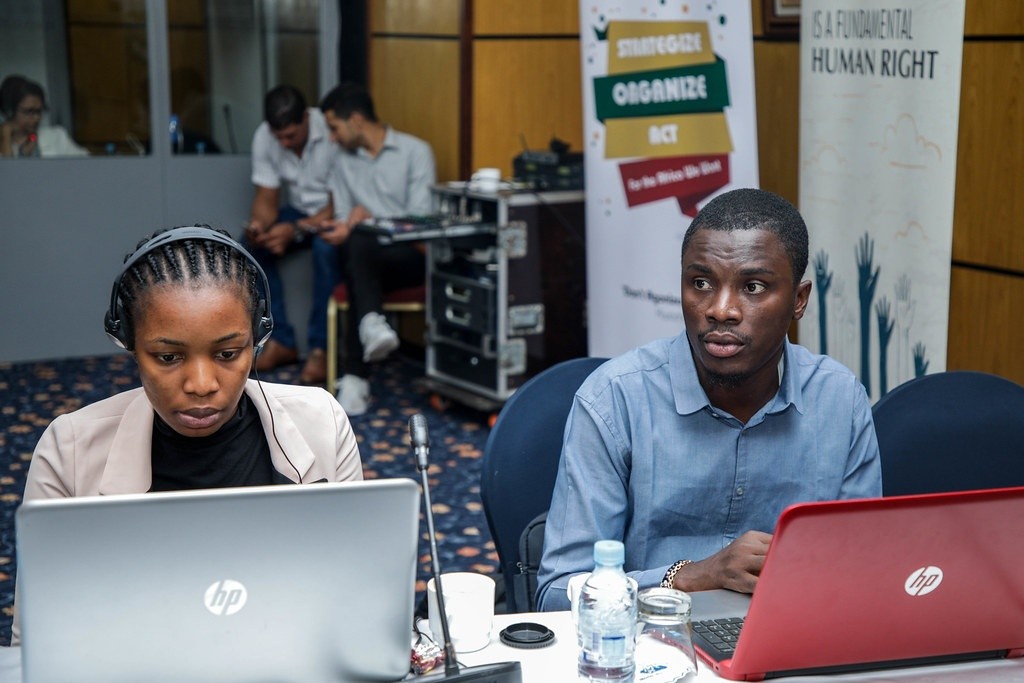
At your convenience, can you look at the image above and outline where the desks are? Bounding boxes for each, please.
[0,609,1024,683]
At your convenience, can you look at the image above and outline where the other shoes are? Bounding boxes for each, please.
[301,349,325,385]
[252,339,299,371]
[359,312,399,362]
[333,374,369,415]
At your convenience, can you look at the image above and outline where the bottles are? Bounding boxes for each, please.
[636,586,699,683]
[577,539,638,683]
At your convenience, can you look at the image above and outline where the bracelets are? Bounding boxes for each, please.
[661,559,692,588]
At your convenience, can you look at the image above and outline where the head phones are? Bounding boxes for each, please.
[105,227,274,357]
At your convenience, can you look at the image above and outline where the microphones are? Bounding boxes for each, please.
[407,414,523,683]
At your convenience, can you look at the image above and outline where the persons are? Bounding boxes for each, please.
[238,82,441,417]
[0,74,91,160]
[534,190,885,611]
[21,223,364,499]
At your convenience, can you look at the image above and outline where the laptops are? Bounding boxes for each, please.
[15,477,422,683]
[647,486,1024,682]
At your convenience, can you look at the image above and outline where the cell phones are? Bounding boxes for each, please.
[240,223,259,238]
[299,222,334,234]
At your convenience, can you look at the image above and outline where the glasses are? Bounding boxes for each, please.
[17,105,43,118]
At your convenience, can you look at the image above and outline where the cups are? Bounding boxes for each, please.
[470,168,500,195]
[427,572,496,653]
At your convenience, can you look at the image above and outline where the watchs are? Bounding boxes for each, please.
[291,221,304,241]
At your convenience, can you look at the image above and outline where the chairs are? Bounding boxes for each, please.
[872,368,1024,497]
[481,355,611,614]
[324,244,428,398]
